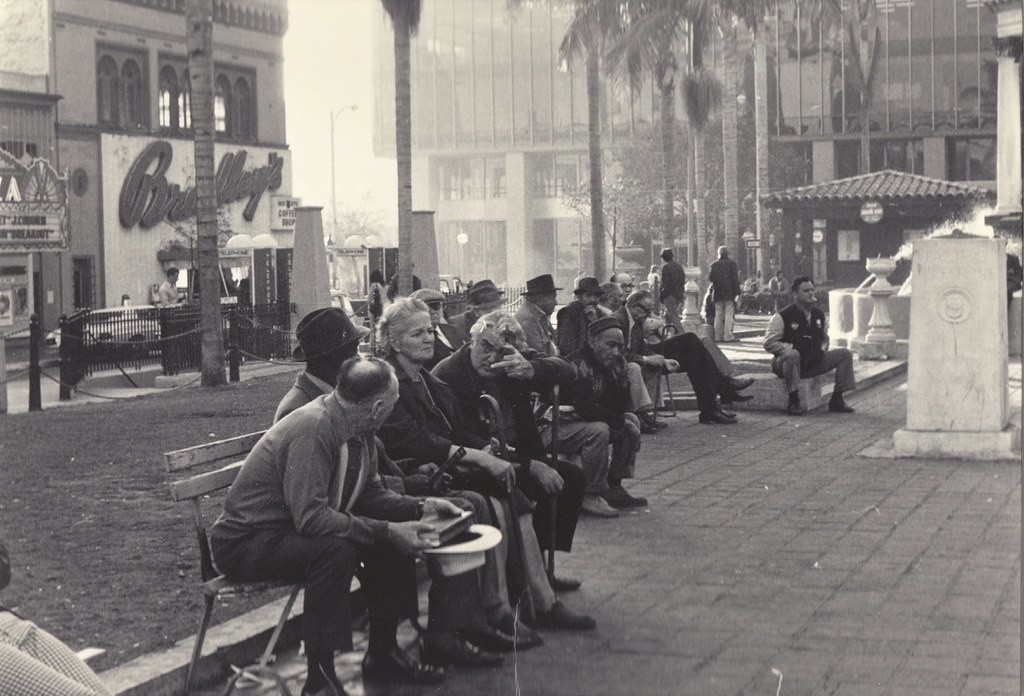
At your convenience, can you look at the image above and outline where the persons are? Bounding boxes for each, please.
[0,540,115,696]
[143,245,789,695]
[996,237,1023,307]
[765,277,856,416]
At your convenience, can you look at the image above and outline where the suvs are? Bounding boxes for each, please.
[45,305,161,363]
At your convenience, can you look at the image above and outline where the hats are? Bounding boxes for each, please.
[661,249,675,257]
[423,522,502,576]
[574,278,606,296]
[465,279,505,295]
[408,288,446,304]
[520,274,564,295]
[292,307,372,362]
[587,317,626,338]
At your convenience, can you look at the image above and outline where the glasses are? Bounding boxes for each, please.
[621,284,635,288]
[427,303,442,310]
[638,303,654,313]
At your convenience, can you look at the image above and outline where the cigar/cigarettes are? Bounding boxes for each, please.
[810,296,817,301]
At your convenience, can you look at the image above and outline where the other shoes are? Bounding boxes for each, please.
[788,397,803,414]
[492,616,543,645]
[582,492,619,517]
[521,601,596,629]
[604,486,648,507]
[547,576,581,591]
[637,412,668,433]
[829,399,854,412]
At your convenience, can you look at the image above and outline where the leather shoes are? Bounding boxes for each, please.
[457,627,533,652]
[723,375,755,391]
[699,406,738,424]
[362,648,446,686]
[720,389,754,403]
[420,629,504,667]
[301,678,346,696]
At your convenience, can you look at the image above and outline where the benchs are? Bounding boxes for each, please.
[163,430,425,696]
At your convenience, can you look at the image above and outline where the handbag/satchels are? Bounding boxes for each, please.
[371,280,383,317]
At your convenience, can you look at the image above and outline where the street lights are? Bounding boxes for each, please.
[741,227,756,280]
[456,229,469,281]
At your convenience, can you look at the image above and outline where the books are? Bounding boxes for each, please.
[416,511,472,547]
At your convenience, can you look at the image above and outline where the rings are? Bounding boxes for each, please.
[501,476,505,481]
